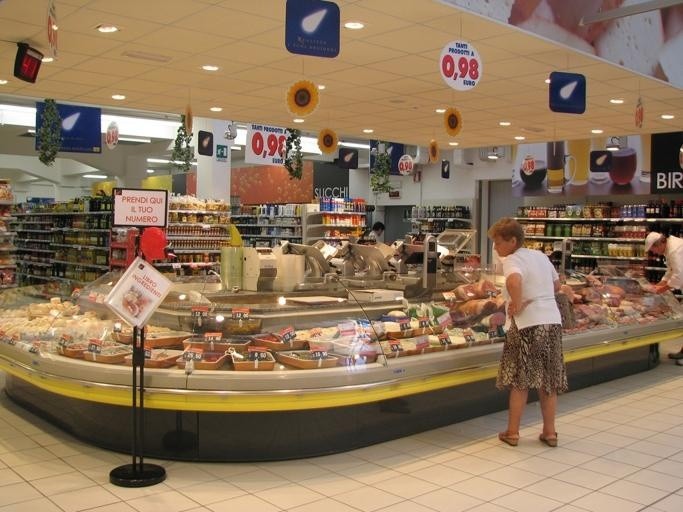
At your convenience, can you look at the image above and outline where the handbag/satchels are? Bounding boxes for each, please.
[555,293,576,329]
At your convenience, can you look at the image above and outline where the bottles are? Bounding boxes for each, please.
[569,258,598,274]
[648,252,666,268]
[15,262,53,277]
[14,240,52,250]
[411,220,463,234]
[54,248,109,266]
[167,226,228,236]
[14,250,53,262]
[54,264,107,282]
[411,203,470,219]
[13,197,113,213]
[167,240,233,250]
[649,223,683,238]
[9,223,52,231]
[648,271,682,294]
[645,200,683,218]
[623,220,647,239]
[178,253,221,262]
[607,242,645,258]
[19,277,46,287]
[16,232,52,239]
[53,215,112,228]
[52,232,109,247]
[16,214,52,222]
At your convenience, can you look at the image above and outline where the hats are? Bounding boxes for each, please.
[645,231,663,252]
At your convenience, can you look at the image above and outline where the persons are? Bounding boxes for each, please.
[488,216,571,450]
[368,221,386,241]
[644,230,683,366]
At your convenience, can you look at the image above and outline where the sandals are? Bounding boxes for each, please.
[540,432,557,447]
[499,431,519,446]
